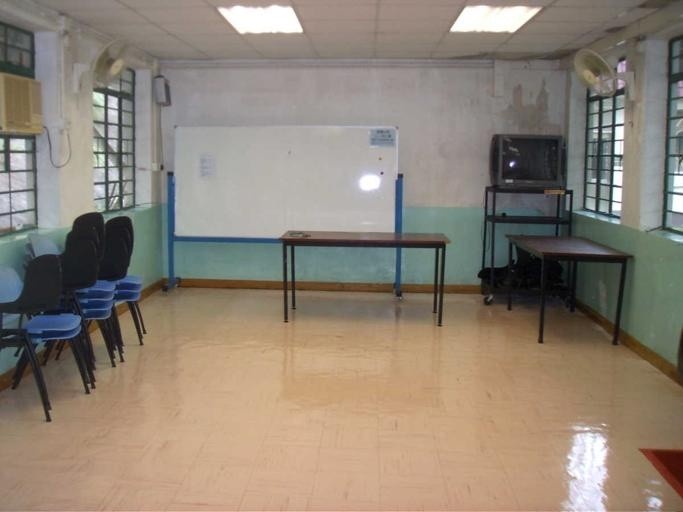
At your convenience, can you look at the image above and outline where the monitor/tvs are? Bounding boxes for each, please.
[489,134,562,189]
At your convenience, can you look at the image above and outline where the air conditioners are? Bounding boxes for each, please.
[0,71,47,139]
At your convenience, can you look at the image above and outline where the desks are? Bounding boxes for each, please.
[505,234,635,351]
[275,229,453,329]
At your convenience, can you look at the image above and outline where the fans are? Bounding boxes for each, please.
[573,48,638,105]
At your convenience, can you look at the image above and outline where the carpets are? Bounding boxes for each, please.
[639,446,682,498]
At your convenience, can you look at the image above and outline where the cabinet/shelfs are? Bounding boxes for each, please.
[480,185,574,308]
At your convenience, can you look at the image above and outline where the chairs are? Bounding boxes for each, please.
[0,211,151,422]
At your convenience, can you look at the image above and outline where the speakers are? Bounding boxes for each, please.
[154,75,169,106]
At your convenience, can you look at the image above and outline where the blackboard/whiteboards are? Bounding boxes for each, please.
[176,127,400,238]
